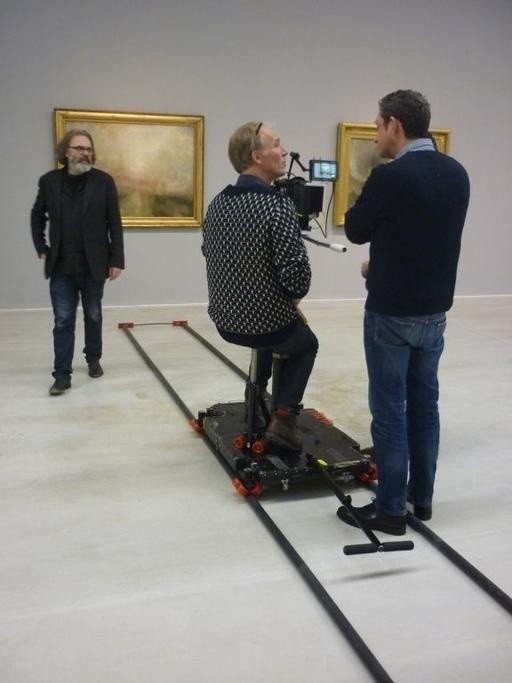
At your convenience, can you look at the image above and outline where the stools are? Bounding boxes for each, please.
[235,343,278,452]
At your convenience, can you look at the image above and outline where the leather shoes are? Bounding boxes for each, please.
[337,497,434,535]
[50,378,74,395]
[88,358,102,378]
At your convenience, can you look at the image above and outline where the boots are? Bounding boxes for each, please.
[244,384,269,430]
[264,409,302,454]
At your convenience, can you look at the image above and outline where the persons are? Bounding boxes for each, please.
[201,121,318,453]
[31,128,125,395]
[337,89,470,535]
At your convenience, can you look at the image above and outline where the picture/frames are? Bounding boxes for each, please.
[53,107,204,227]
[332,123,450,227]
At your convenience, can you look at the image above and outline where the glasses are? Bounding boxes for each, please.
[66,144,95,153]
